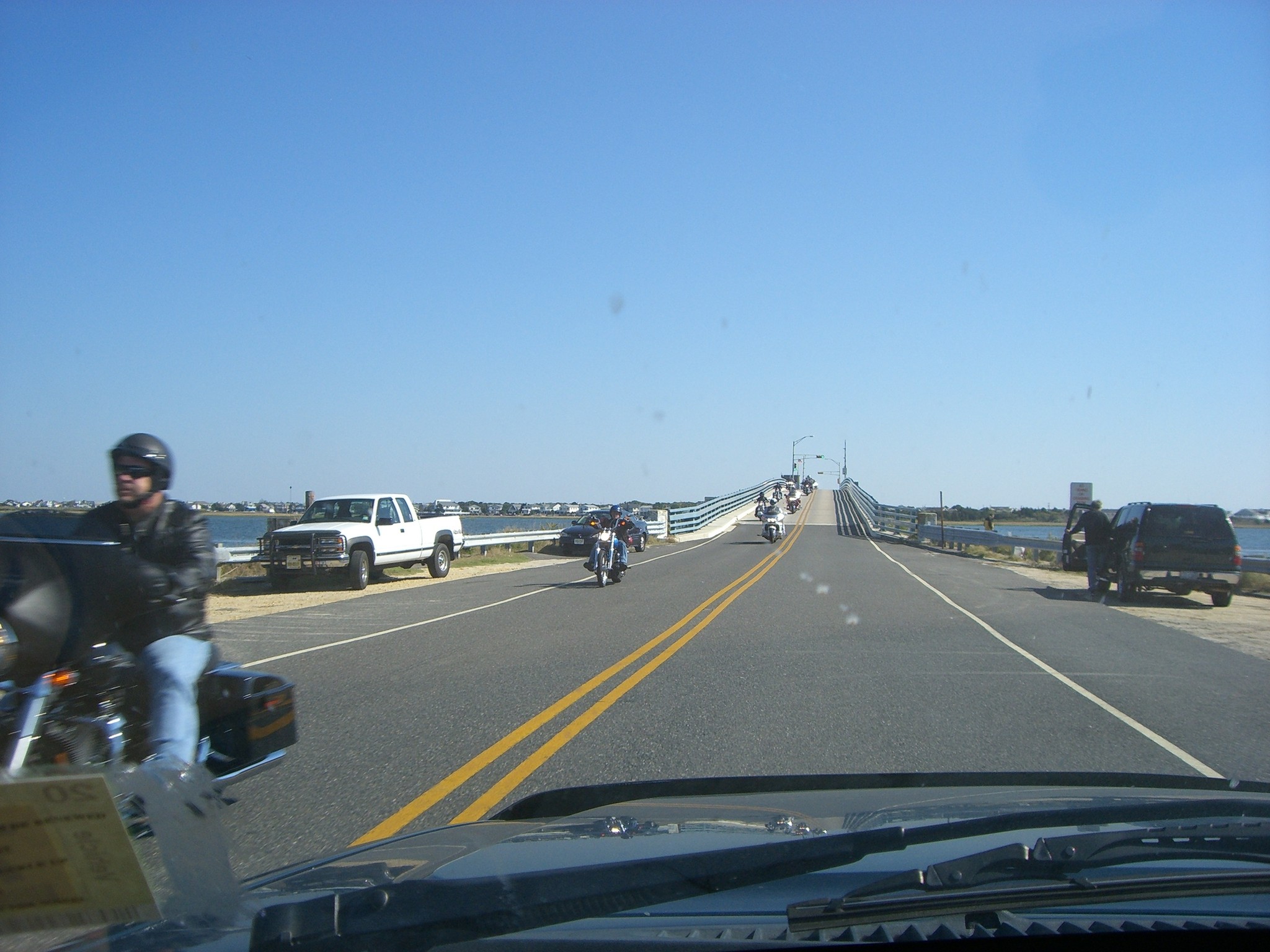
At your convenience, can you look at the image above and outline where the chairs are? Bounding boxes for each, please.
[352,503,367,520]
[378,506,391,521]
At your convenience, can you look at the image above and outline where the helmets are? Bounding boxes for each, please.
[610,505,622,515]
[770,498,778,507]
[760,492,764,496]
[112,433,170,477]
[807,475,811,478]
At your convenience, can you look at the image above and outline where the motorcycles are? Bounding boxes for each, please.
[802,483,812,496]
[584,512,633,588]
[0,506,303,838]
[756,511,787,544]
[784,491,802,514]
[752,498,770,520]
[773,487,783,502]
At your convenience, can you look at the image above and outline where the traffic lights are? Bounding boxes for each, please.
[816,455,824,458]
[794,463,797,471]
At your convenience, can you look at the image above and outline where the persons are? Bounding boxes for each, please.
[762,498,786,536]
[584,505,633,572]
[802,475,813,493]
[754,492,769,517]
[599,517,605,526]
[786,478,797,490]
[984,513,995,530]
[786,488,801,507]
[773,482,783,499]
[1064,500,1112,597]
[81,433,218,764]
[1113,517,1139,562]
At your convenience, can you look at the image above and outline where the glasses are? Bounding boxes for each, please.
[113,463,153,479]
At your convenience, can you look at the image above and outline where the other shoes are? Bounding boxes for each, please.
[619,562,627,572]
[1088,587,1097,596]
[583,562,594,572]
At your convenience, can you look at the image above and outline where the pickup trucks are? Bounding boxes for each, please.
[256,493,467,590]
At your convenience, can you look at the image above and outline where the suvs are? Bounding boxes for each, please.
[1061,502,1242,608]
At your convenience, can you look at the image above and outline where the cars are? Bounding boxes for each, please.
[558,508,649,552]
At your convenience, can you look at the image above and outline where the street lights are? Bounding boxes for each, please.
[792,435,814,481]
[825,458,841,489]
